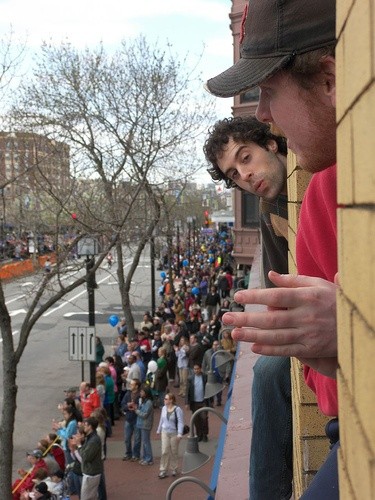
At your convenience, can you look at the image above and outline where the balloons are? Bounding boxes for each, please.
[209,257,213,263]
[108,314,118,327]
[192,287,198,294]
[160,272,165,277]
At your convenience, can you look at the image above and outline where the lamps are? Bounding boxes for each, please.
[204,350,236,398]
[215,326,238,368]
[180,407,228,474]
[234,279,248,295]
[229,301,246,313]
[165,475,215,500]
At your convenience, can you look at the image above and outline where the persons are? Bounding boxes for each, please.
[0,229,57,260]
[133,386,153,464]
[185,363,209,443]
[121,379,143,462]
[12,381,107,500]
[43,258,51,276]
[156,393,184,478]
[138,226,247,397]
[202,340,230,408]
[96,317,168,436]
[206,0,342,500]
[107,253,112,269]
[203,117,293,500]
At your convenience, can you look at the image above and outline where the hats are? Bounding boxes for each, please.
[63,388,76,392]
[204,0,336,98]
[48,470,64,478]
[35,482,48,494]
[27,449,43,458]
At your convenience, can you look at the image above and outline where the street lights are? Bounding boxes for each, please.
[77,230,103,389]
[145,225,158,317]
[186,216,193,266]
[174,218,182,278]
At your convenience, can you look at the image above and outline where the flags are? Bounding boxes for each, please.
[182,260,188,267]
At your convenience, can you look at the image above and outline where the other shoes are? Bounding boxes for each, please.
[172,471,176,476]
[158,472,167,478]
[130,457,140,462]
[141,461,153,466]
[123,457,130,460]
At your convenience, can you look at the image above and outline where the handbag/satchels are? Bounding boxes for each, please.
[183,425,189,435]
